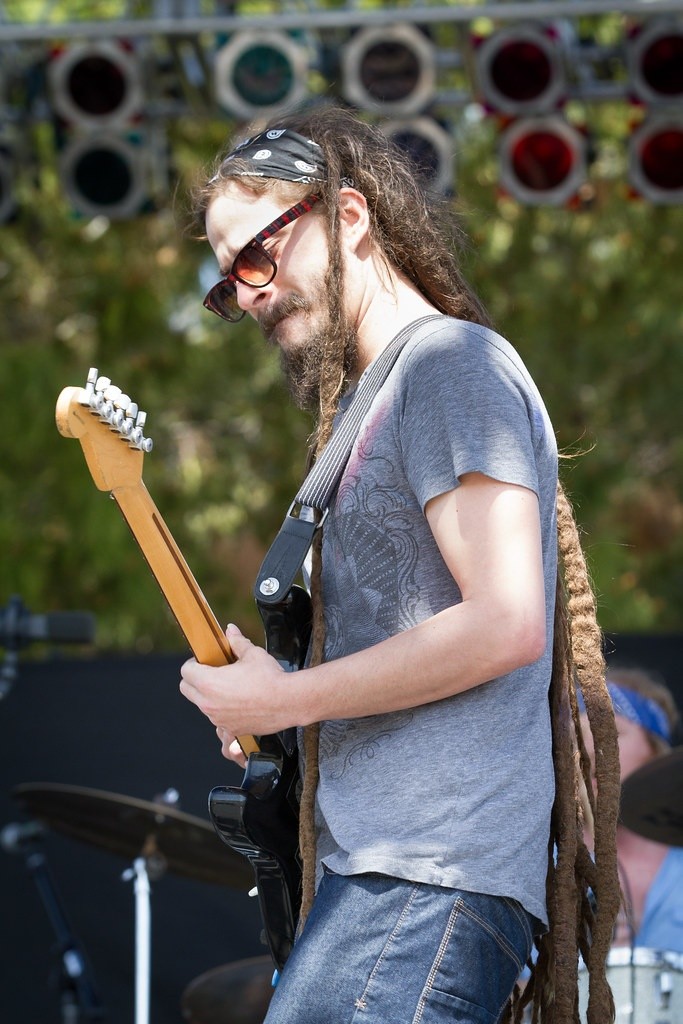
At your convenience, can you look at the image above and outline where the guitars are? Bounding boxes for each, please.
[54,366,316,979]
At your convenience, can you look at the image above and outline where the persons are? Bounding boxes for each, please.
[510,665,683,1024]
[178,106,629,1024]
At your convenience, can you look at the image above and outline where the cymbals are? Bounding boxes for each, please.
[617,746,683,847]
[13,780,255,896]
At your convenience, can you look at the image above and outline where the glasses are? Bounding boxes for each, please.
[201,190,326,323]
